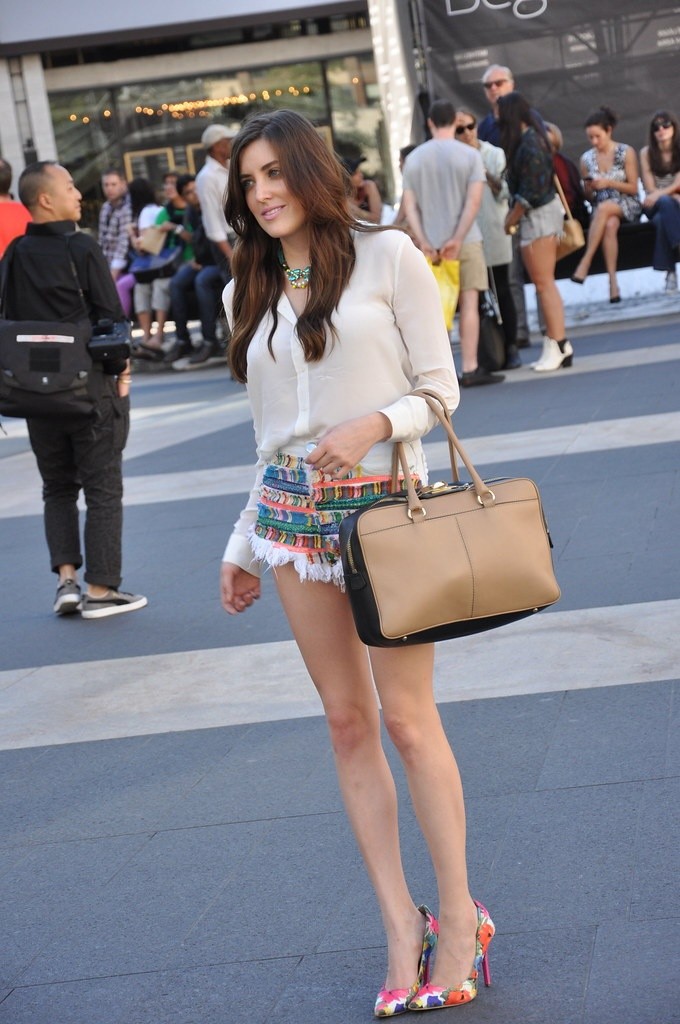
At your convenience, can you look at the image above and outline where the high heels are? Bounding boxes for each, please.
[530,335,573,372]
[374,905,438,1017]
[407,901,496,1009]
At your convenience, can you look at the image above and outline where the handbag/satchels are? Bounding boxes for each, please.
[0,318,96,416]
[557,219,585,260]
[129,248,182,281]
[337,391,563,646]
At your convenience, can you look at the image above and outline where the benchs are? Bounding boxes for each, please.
[506,215,680,286]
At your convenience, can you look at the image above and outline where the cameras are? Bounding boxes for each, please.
[87,319,131,375]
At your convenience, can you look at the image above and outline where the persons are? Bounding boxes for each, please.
[402,66,590,387]
[0,157,32,259]
[0,160,149,618]
[336,144,423,223]
[638,111,680,295]
[95,124,245,371]
[571,105,643,304]
[220,109,495,1018]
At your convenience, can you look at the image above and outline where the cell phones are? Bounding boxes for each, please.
[583,177,592,181]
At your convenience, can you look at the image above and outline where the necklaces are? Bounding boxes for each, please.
[277,248,314,291]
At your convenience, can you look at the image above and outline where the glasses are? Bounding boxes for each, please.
[482,79,508,88]
[455,122,475,134]
[653,120,671,132]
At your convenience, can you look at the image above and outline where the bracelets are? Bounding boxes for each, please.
[174,224,184,236]
[116,371,133,383]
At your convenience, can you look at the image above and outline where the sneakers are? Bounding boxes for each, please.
[80,590,147,618]
[53,579,81,614]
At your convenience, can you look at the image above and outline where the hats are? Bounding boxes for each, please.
[343,156,366,173]
[202,124,237,148]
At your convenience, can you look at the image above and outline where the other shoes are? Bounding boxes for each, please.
[609,289,621,302]
[167,336,193,362]
[193,341,219,362]
[573,269,585,284]
[462,367,505,386]
[666,271,677,293]
[141,331,165,346]
